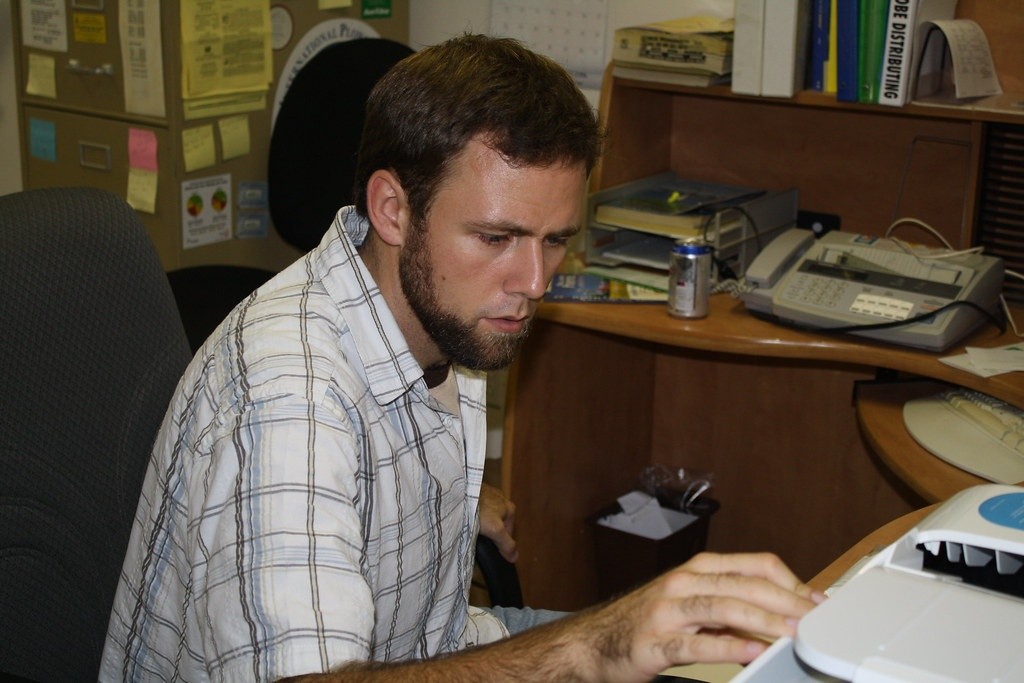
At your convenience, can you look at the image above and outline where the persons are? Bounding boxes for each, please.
[91,31,825,683]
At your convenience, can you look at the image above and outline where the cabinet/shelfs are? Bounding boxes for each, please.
[500,60,1024,683]
[9,0,409,272]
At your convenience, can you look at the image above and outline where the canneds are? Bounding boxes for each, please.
[668,238,711,319]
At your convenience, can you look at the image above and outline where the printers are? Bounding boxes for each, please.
[722,483,1024,683]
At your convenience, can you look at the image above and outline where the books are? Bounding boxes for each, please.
[615,0,957,109]
[582,177,771,299]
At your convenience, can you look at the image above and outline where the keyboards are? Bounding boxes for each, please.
[902,387,1024,484]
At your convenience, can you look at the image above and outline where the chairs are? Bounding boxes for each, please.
[0,190,195,682]
